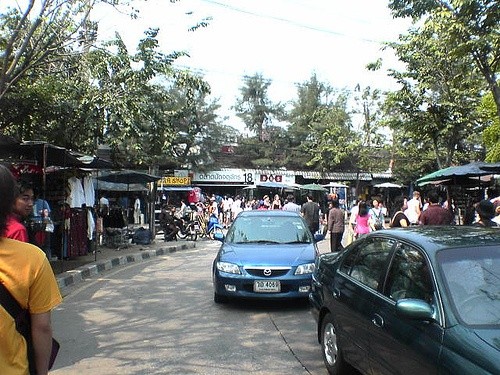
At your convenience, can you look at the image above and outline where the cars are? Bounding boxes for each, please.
[212,209,325,301]
[309,226,500,375]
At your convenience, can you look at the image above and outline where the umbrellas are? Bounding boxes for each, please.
[322,183,348,192]
[373,182,400,211]
[414,162,500,225]
[96,169,162,190]
[299,182,330,193]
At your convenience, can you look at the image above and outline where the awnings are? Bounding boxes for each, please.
[0,141,114,262]
[242,181,301,199]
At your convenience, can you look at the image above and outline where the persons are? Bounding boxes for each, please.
[33,188,51,218]
[473,198,499,226]
[419,190,456,226]
[404,191,423,225]
[0,164,63,375]
[301,195,320,238]
[240,191,302,212]
[328,198,344,254]
[98,192,109,227]
[349,189,390,239]
[132,193,140,225]
[2,180,33,244]
[155,193,242,240]
[390,195,411,227]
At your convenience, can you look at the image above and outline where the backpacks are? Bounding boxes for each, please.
[369,208,382,231]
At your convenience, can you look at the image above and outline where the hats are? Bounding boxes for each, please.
[476,200,495,219]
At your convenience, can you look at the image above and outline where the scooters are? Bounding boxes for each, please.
[175,215,201,241]
[197,212,224,240]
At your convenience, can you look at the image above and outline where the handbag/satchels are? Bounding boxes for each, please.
[15,309,60,375]
